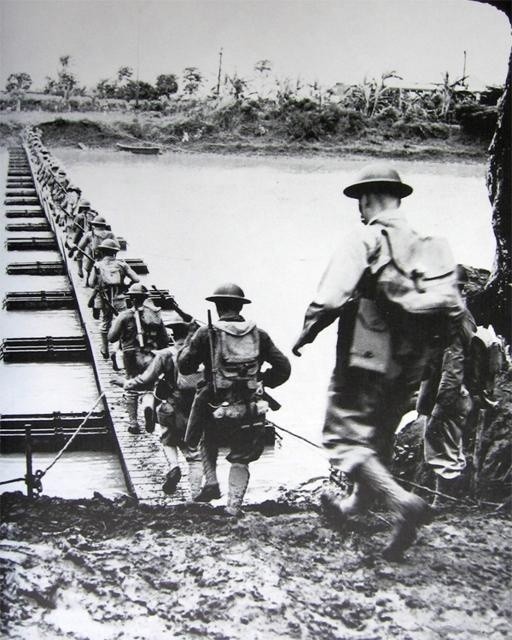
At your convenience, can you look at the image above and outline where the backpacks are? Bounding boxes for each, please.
[365,217,464,317]
[213,322,262,392]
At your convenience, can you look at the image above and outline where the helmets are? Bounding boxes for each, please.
[205,282,251,304]
[51,164,153,295]
[342,163,413,199]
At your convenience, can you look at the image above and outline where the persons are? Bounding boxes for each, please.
[108,317,207,501]
[24,122,116,287]
[414,265,479,509]
[175,282,292,517]
[87,239,141,359]
[294,164,463,559]
[106,282,169,433]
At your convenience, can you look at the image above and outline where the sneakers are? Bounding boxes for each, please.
[381,496,434,558]
[194,483,220,501]
[163,467,181,494]
[127,405,156,434]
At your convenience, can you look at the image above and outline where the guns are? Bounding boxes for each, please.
[94,288,119,316]
[55,180,95,264]
[152,284,200,329]
[166,297,281,411]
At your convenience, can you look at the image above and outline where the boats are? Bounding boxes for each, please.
[116,143,160,155]
